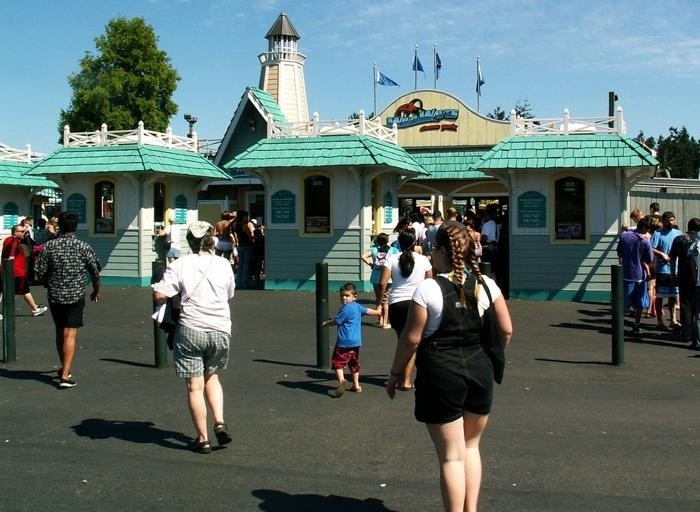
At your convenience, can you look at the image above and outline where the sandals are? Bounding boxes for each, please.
[214,422,232,446]
[187,439,211,454]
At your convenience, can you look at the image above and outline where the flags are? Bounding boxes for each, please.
[475,67,485,97]
[376,70,401,87]
[436,52,442,80]
[412,51,424,73]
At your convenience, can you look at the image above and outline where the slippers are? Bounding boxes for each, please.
[351,383,363,393]
[334,380,349,397]
[402,382,414,391]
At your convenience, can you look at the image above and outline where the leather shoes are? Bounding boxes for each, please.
[645,308,657,320]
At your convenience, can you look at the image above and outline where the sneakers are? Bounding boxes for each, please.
[31,304,48,317]
[656,321,681,332]
[58,367,76,388]
[633,324,645,336]
[376,321,392,329]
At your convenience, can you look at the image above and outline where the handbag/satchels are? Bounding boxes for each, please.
[162,240,172,249]
[483,306,505,384]
[158,292,180,333]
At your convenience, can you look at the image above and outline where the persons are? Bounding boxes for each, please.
[212,209,264,287]
[360,202,501,391]
[385,220,512,512]
[34,210,103,389]
[616,200,700,350]
[159,216,181,264]
[0,215,58,320]
[150,220,237,455]
[320,283,383,396]
